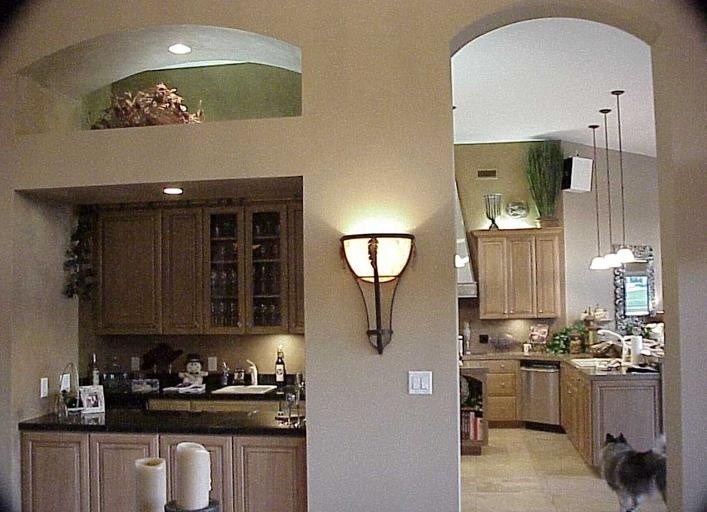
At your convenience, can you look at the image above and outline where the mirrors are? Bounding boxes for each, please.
[612,244,655,330]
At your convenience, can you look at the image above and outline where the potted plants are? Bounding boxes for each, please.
[524,139,565,227]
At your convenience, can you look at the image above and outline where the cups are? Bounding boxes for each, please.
[523,343,531,356]
[217,367,229,386]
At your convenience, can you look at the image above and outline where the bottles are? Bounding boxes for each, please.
[86,352,100,386]
[212,215,280,329]
[274,344,286,387]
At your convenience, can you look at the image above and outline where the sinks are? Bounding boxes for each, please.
[211,383,278,395]
[570,357,621,367]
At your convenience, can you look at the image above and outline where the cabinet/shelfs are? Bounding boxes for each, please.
[559,358,658,477]
[18,431,306,512]
[161,207,204,335]
[204,204,288,334]
[93,206,161,334]
[473,227,562,320]
[287,202,305,335]
[483,361,522,429]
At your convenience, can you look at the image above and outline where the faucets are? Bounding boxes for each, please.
[596,329,628,362]
[244,359,259,386]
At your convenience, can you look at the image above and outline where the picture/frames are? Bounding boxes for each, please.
[79,385,105,414]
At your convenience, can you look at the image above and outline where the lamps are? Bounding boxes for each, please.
[611,90,635,263]
[339,233,415,353]
[589,125,609,270]
[600,109,621,267]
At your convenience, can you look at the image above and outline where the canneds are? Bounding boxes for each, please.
[569,333,582,354]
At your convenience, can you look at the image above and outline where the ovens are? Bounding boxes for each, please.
[517,361,560,427]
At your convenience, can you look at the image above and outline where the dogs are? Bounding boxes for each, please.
[600,431,667,512]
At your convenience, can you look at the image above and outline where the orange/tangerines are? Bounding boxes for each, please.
[558,318,584,333]
[544,332,569,355]
